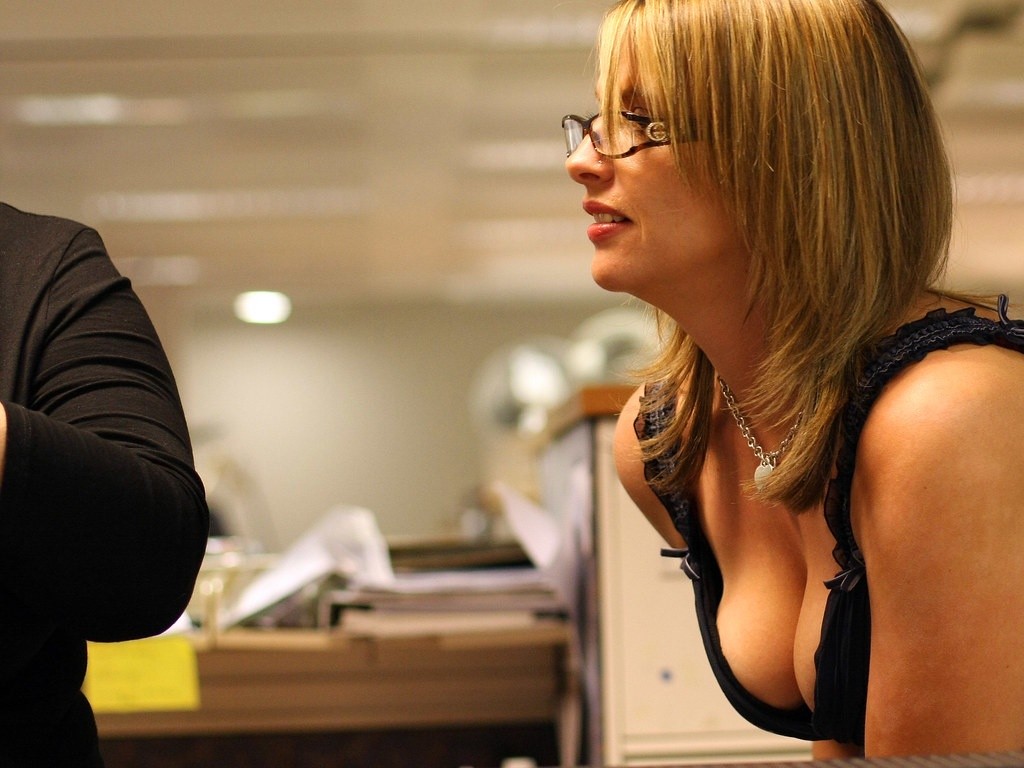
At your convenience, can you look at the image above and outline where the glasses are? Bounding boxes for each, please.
[561,108,732,159]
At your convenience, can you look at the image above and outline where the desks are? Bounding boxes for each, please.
[78,612,588,768]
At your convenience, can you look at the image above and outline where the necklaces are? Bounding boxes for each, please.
[716,373,801,491]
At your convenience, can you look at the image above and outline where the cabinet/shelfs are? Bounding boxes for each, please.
[539,384,814,767]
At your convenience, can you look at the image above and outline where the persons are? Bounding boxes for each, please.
[0,202,209,768]
[563,0,1024,762]
